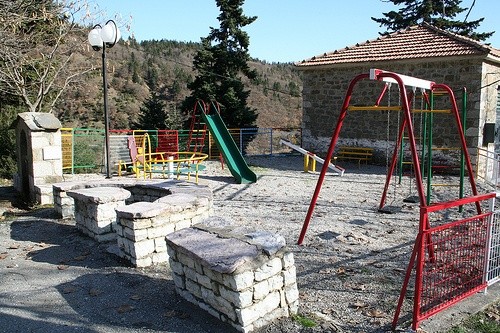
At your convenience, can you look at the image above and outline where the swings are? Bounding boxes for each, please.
[378,86,402,214]
[403,92,427,203]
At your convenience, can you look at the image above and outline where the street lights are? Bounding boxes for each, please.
[88,20,121,178]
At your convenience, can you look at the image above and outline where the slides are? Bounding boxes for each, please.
[197,101,257,183]
